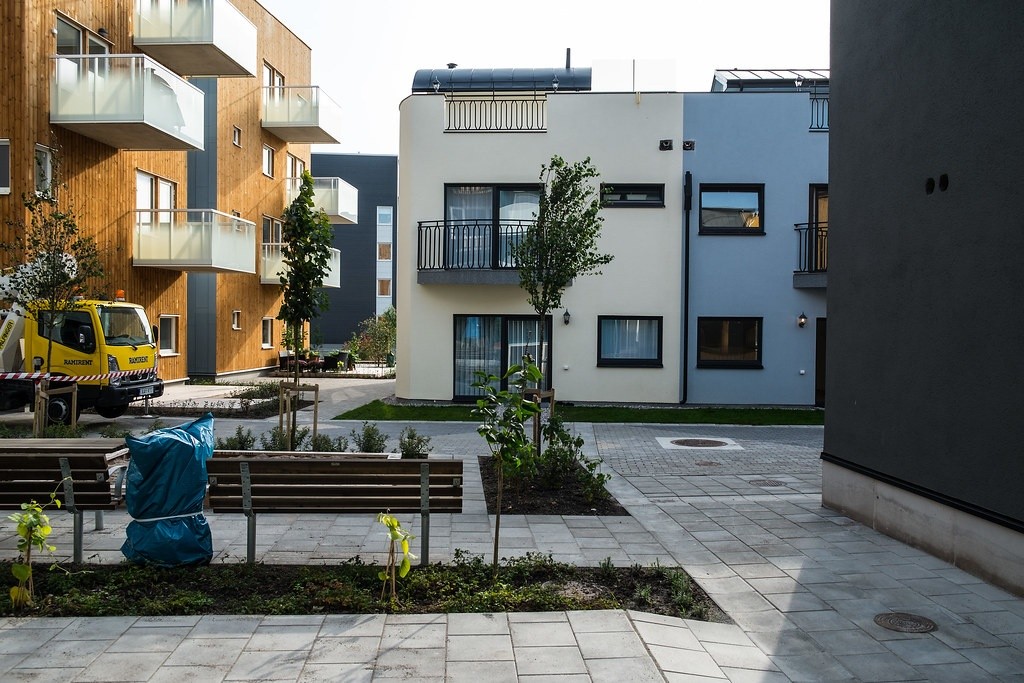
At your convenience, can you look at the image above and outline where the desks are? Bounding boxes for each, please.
[0,439,128,530]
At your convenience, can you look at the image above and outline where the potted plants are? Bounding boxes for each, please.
[309,351,318,361]
[399,425,433,460]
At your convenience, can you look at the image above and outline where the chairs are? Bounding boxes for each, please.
[321,352,348,372]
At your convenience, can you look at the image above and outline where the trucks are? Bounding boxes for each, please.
[0,252,164,429]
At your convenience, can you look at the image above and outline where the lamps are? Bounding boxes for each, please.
[795,74,804,92]
[432,75,441,94]
[552,74,561,94]
[564,308,571,325]
[798,311,808,327]
[99,27,110,37]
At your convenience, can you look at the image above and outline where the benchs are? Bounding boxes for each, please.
[0,453,127,570]
[207,458,464,568]
[277,350,295,372]
